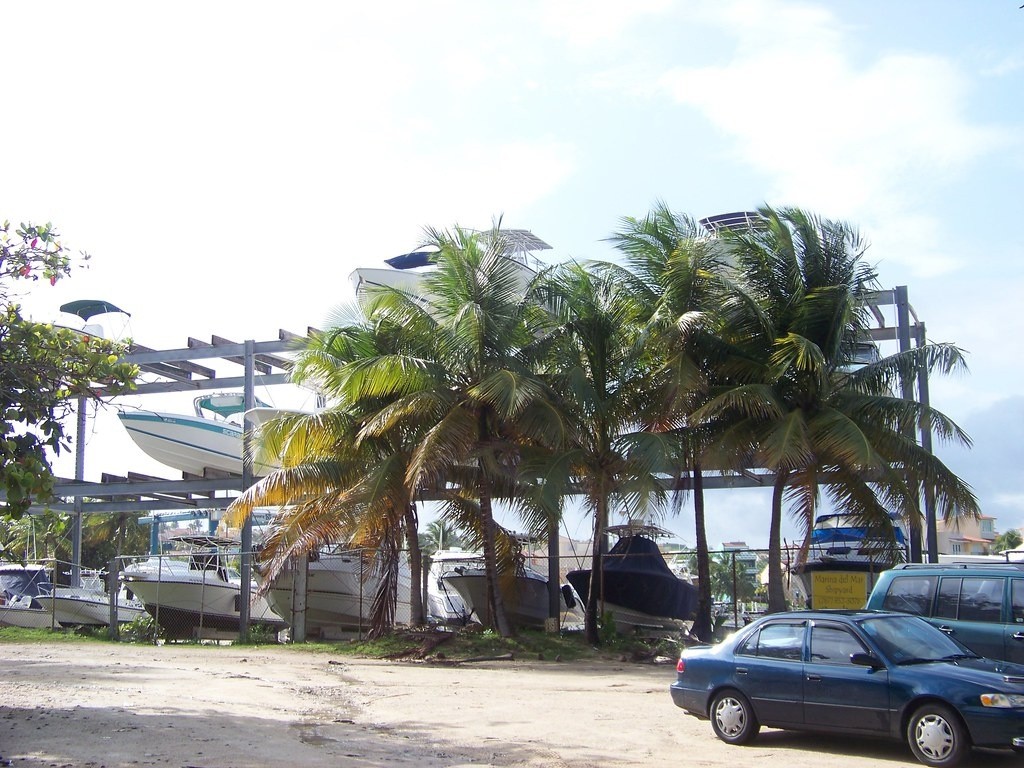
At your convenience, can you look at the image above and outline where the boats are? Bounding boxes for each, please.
[20,299,133,366]
[791,509,908,610]
[683,212,774,296]
[345,225,555,332]
[564,520,717,641]
[1,391,581,639]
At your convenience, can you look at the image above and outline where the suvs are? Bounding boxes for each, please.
[865,561,1024,668]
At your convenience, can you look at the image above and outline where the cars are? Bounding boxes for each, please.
[670,607,1024,768]
